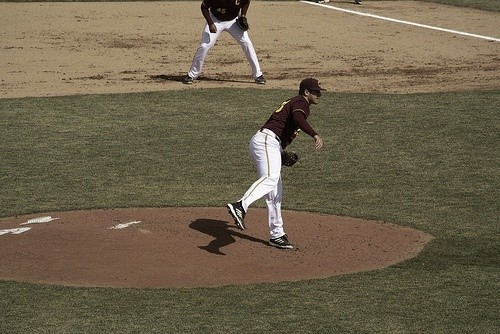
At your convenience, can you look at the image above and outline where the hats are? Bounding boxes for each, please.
[300,78,327,90]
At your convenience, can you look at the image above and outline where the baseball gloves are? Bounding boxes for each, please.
[235,16,249,31]
[281,150,299,167]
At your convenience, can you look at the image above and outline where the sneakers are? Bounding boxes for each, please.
[227,203,245,230]
[255,75,266,83]
[184,76,193,83]
[269,234,294,249]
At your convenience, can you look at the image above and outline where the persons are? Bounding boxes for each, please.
[226,78,327,250]
[183,0,266,85]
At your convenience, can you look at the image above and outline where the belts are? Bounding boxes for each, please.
[259,129,282,144]
